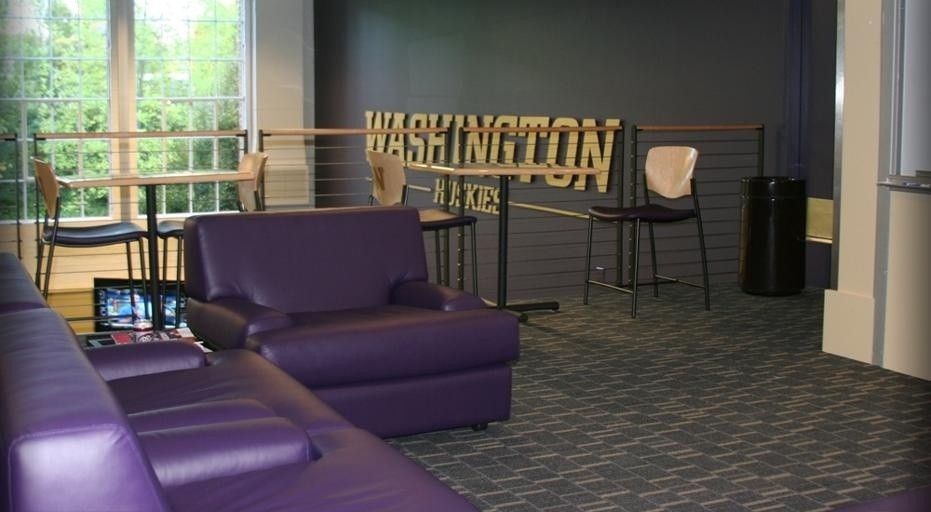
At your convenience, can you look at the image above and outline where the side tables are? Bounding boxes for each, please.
[87,329,225,355]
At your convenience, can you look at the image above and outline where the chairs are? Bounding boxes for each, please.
[363,149,480,296]
[583,146,711,318]
[32,158,151,321]
[156,151,270,328]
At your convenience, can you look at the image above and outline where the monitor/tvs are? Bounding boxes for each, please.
[92,276,189,331]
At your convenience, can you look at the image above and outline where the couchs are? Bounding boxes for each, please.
[0,252,479,512]
[183,204,518,441]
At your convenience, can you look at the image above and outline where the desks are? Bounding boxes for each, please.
[403,160,598,321]
[54,171,258,328]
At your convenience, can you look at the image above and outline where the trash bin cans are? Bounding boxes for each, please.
[738,178,806,295]
